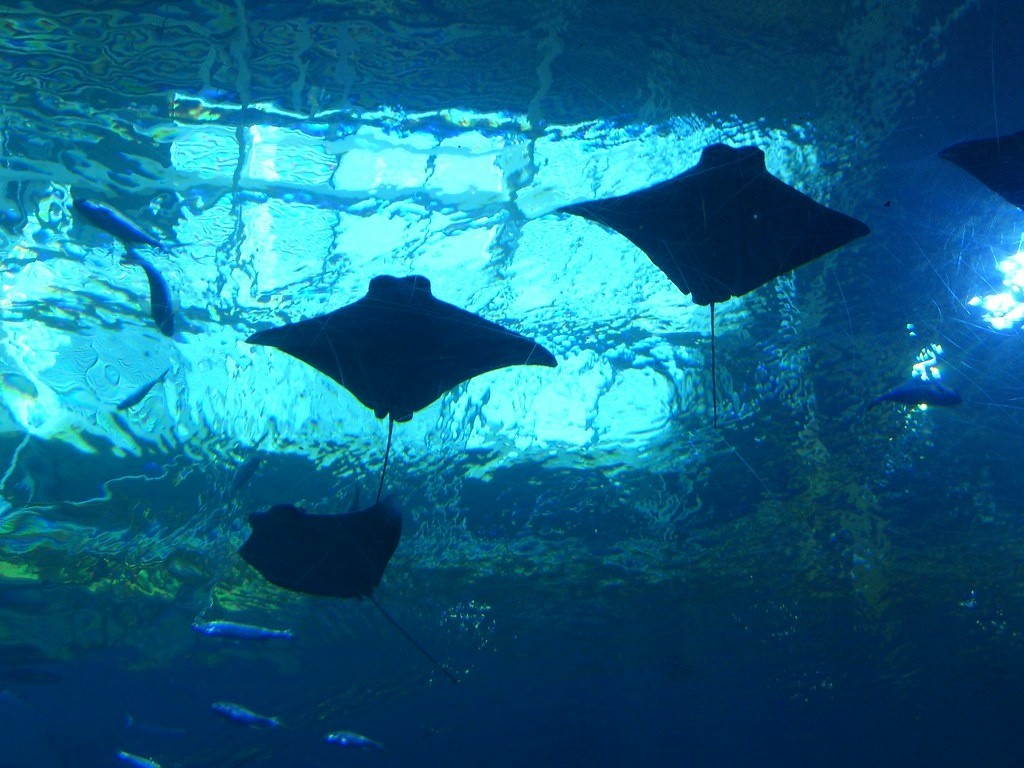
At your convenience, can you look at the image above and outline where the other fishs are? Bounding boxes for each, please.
[936,129,1024,214]
[210,701,289,734]
[113,748,162,768]
[190,621,297,642]
[119,244,175,338]
[228,451,263,502]
[557,141,872,431]
[238,503,460,684]
[115,366,171,410]
[72,196,193,259]
[326,729,389,753]
[866,373,961,411]
[245,275,557,509]
